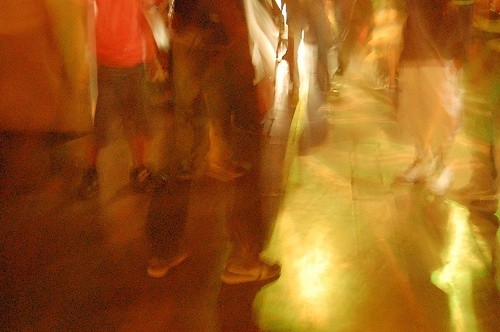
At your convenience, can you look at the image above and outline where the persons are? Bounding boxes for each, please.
[280,0,331,108]
[1,0,94,181]
[73,1,171,196]
[333,0,374,76]
[147,0,281,284]
[398,0,459,195]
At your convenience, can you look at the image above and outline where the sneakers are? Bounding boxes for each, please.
[77,168,100,201]
[146,249,193,278]
[128,166,157,194]
[220,254,281,284]
